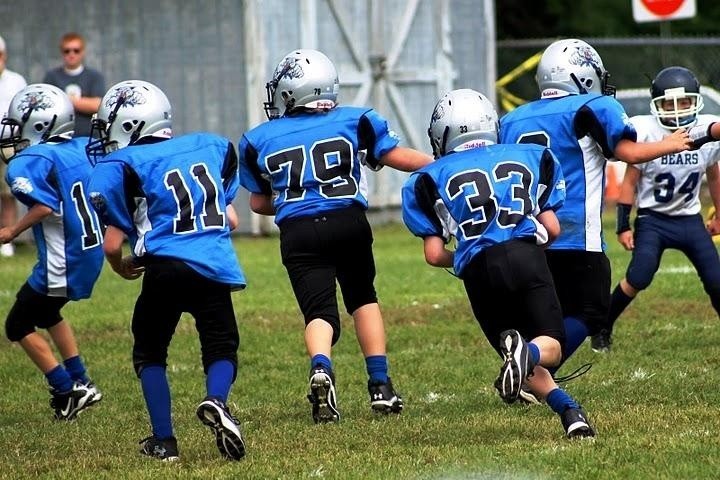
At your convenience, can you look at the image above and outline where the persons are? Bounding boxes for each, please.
[493,38,696,409]
[402,88,594,438]
[0,82,105,422]
[586,68,720,353]
[44,32,109,137]
[89,79,253,460]
[0,33,33,259]
[240,50,436,424]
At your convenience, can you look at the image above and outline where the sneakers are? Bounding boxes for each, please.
[497,329,534,405]
[307,363,340,425]
[493,363,592,406]
[368,377,404,414]
[591,327,613,353]
[85,380,103,409]
[139,433,179,463]
[560,403,594,439]
[0,240,15,257]
[196,396,246,461]
[49,380,95,421]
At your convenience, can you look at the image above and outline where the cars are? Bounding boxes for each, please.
[603,85,720,189]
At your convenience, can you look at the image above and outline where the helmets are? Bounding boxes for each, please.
[428,88,501,158]
[0,82,76,164]
[535,38,617,100]
[263,48,339,120]
[85,79,172,166]
[650,66,705,133]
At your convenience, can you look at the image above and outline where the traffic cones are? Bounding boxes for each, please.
[605,165,622,201]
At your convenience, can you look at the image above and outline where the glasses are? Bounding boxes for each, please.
[64,49,80,53]
